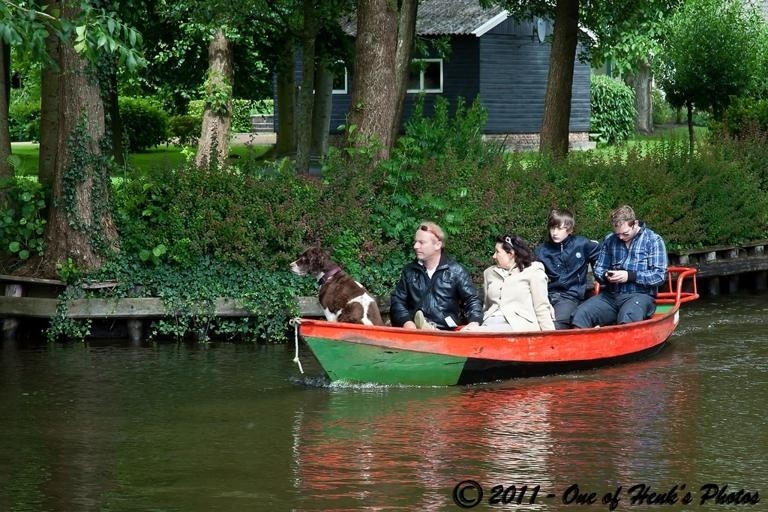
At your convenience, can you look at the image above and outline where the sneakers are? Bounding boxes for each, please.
[414,309,441,330]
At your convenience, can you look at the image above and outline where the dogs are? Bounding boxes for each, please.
[289,238,385,327]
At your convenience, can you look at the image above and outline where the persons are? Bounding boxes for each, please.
[402,234,556,333]
[530,207,601,328]
[570,204,670,329]
[389,223,483,329]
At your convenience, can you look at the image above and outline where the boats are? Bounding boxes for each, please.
[290,262,700,389]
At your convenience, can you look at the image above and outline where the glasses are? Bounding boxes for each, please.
[614,225,635,237]
[416,224,444,242]
